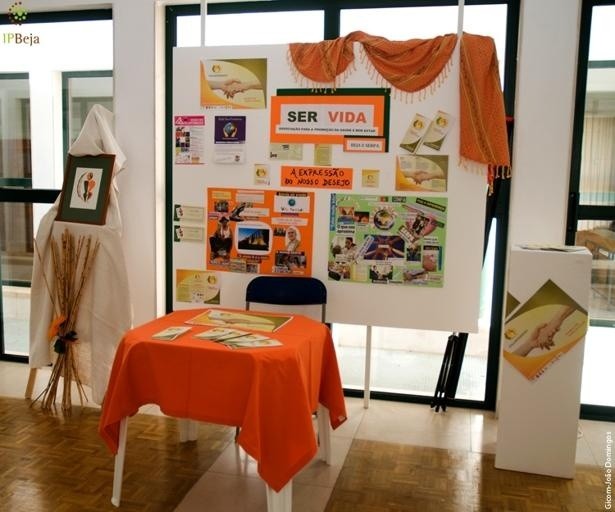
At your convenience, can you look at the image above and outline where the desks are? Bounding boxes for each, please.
[95,307,348,512]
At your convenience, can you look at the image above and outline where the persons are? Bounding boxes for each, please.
[400,170,430,182]
[537,305,577,350]
[413,171,447,185]
[223,79,264,99]
[208,216,233,264]
[224,318,277,327]
[222,122,239,138]
[287,228,301,250]
[330,206,441,285]
[207,315,248,325]
[511,322,560,357]
[208,79,244,96]
[275,249,306,273]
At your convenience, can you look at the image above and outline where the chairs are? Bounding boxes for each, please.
[234,275,328,448]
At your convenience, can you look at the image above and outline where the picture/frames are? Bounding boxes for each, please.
[53,153,115,226]
